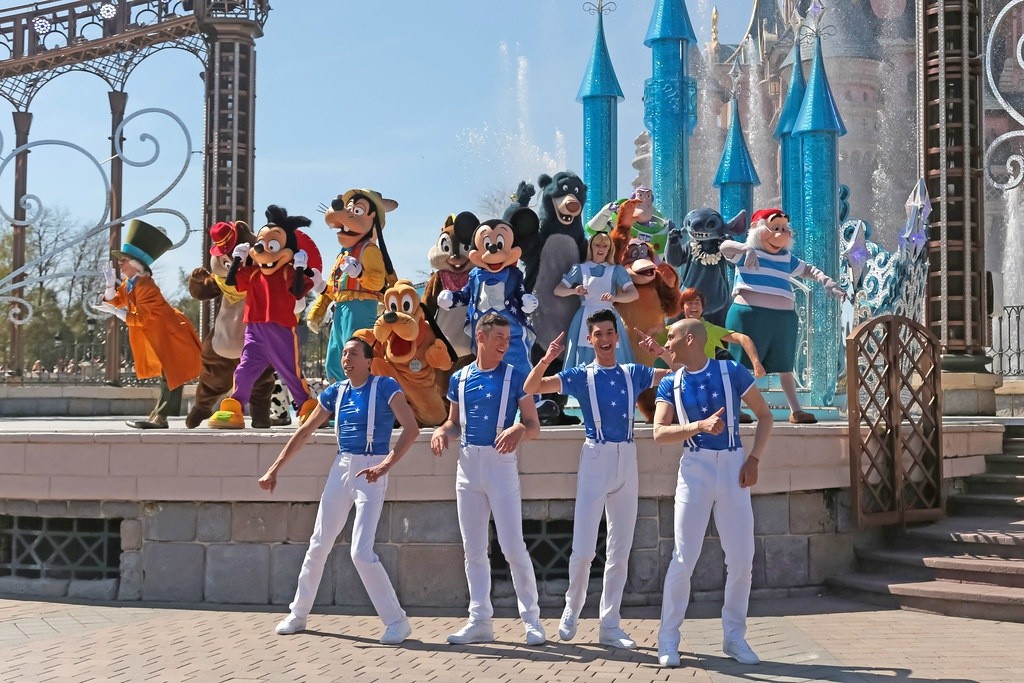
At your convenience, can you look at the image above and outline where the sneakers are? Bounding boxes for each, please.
[659,641,680,666]
[521,618,546,645]
[599,626,636,649]
[723,639,759,664]
[276,612,305,634]
[559,601,584,641]
[447,619,494,643]
[380,618,411,645]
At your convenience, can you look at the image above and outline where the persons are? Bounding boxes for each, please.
[523,308,685,650]
[89,217,204,431]
[258,337,419,647]
[652,318,774,668]
[554,229,640,425]
[428,314,546,646]
[667,287,768,378]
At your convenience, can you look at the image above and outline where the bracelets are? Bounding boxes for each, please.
[657,346,665,355]
[540,357,551,365]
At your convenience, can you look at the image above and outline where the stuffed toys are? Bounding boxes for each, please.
[306,189,399,428]
[663,204,751,424]
[500,169,588,426]
[586,186,674,264]
[205,204,329,430]
[419,210,480,430]
[610,196,678,424]
[437,218,558,420]
[352,278,453,429]
[720,207,846,425]
[185,220,273,429]
